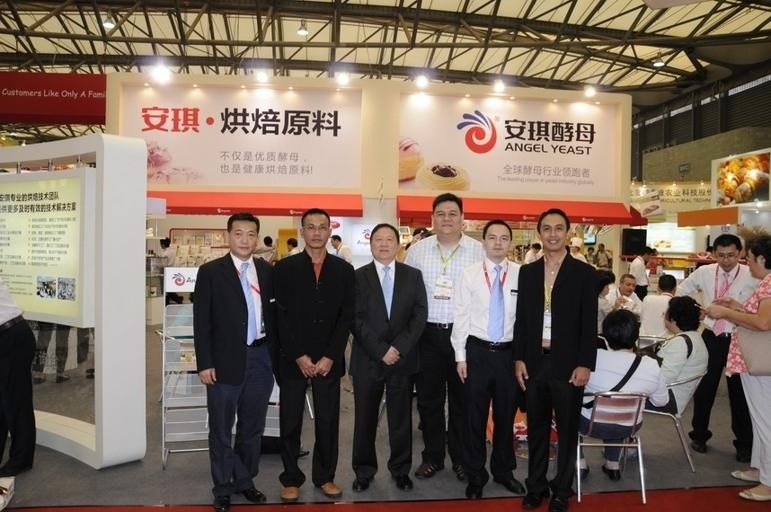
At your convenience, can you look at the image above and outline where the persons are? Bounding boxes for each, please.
[192,211,275,512]
[32,320,70,385]
[673,233,762,463]
[629,247,653,302]
[606,274,642,311]
[601,295,709,461]
[0,278,37,476]
[397,227,429,262]
[254,236,276,265]
[596,269,628,335]
[573,309,670,482]
[84,327,94,378]
[286,237,304,257]
[583,247,597,267]
[570,237,587,263]
[404,193,485,482]
[510,207,599,512]
[331,235,353,266]
[448,218,525,499]
[159,237,177,295]
[347,222,429,493]
[596,243,613,271]
[646,248,658,275]
[705,235,771,502]
[632,274,677,349]
[270,206,355,503]
[524,243,542,265]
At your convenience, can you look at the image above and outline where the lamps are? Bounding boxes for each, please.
[653,50,664,66]
[103,9,115,29]
[296,20,308,36]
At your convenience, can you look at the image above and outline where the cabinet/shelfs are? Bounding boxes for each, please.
[162,267,209,469]
[146,198,167,326]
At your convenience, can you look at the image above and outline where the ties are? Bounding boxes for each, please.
[381,266,393,315]
[486,265,505,340]
[240,263,258,347]
[712,272,729,337]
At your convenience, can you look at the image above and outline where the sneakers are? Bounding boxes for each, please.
[453,464,465,480]
[415,461,444,479]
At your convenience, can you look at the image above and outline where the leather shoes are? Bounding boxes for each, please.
[549,491,570,511]
[33,377,46,384]
[0,459,32,478]
[601,463,621,481]
[280,486,300,501]
[213,495,230,511]
[522,485,550,510]
[691,436,708,452]
[493,472,525,494]
[575,464,590,480]
[321,481,342,497]
[465,472,489,498]
[233,486,267,503]
[352,473,374,491]
[738,488,771,501]
[56,374,70,383]
[730,470,761,482]
[391,473,413,491]
[736,448,751,463]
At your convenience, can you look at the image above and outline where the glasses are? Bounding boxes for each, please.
[745,255,752,262]
[302,224,329,233]
[717,252,740,259]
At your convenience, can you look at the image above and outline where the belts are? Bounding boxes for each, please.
[469,336,513,351]
[0,315,24,333]
[252,336,266,347]
[542,346,551,355]
[425,322,453,331]
[719,331,732,338]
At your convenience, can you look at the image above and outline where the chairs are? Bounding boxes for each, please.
[155,329,194,402]
[643,370,708,472]
[638,335,666,352]
[555,392,650,504]
